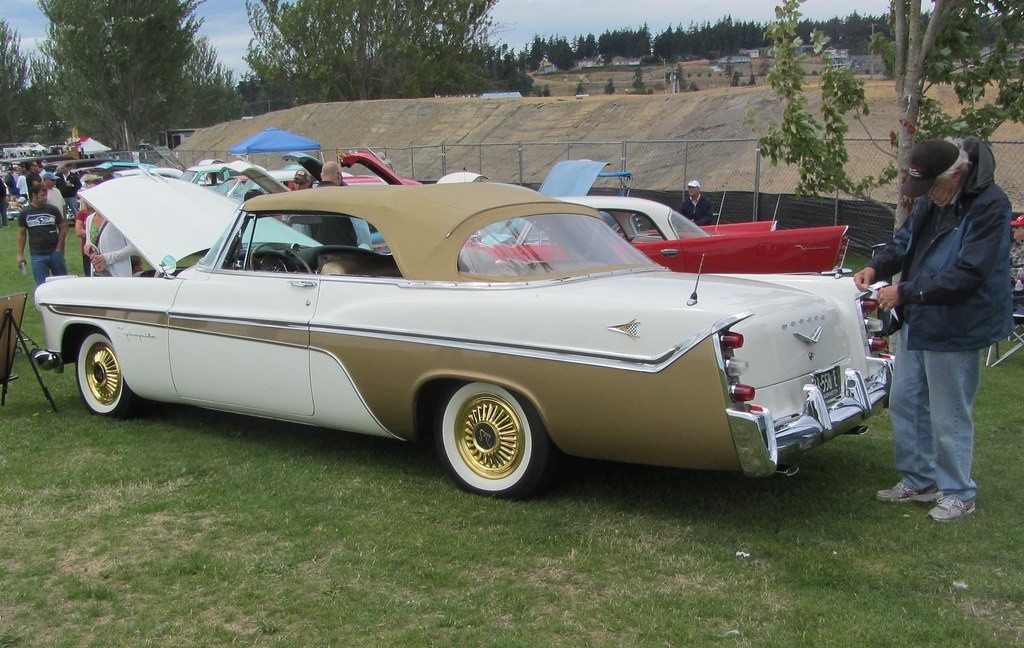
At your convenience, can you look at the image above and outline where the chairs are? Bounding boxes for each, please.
[321,255,380,276]
[984,290,1024,367]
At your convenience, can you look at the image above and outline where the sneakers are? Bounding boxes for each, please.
[927,493,977,522]
[877,477,943,502]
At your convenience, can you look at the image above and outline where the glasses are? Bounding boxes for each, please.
[337,172,343,181]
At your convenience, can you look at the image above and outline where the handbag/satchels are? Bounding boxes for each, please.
[6,187,9,195]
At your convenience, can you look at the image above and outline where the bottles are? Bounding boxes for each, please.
[89,248,103,272]
[20,262,28,276]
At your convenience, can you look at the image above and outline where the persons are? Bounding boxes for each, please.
[854,136,1014,523]
[42,172,66,233]
[130,255,143,274]
[55,163,82,222]
[678,180,713,225]
[17,185,67,286]
[0,160,46,226]
[83,179,140,276]
[282,170,315,235]
[1010,215,1024,312]
[378,152,395,174]
[75,201,96,275]
[309,162,360,273]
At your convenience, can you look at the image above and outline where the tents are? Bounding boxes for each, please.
[78,138,111,158]
[73,137,88,148]
[227,128,323,171]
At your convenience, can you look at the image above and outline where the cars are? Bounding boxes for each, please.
[372,195,854,279]
[34,171,893,501]
[72,142,611,252]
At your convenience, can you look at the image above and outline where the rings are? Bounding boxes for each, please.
[879,303,885,308]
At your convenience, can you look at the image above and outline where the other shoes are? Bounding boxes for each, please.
[3,226,9,228]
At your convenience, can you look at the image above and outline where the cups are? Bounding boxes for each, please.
[66,181,70,185]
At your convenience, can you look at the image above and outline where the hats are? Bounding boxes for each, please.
[294,170,308,181]
[687,180,700,188]
[1010,214,1024,225]
[43,172,60,180]
[902,140,959,199]
